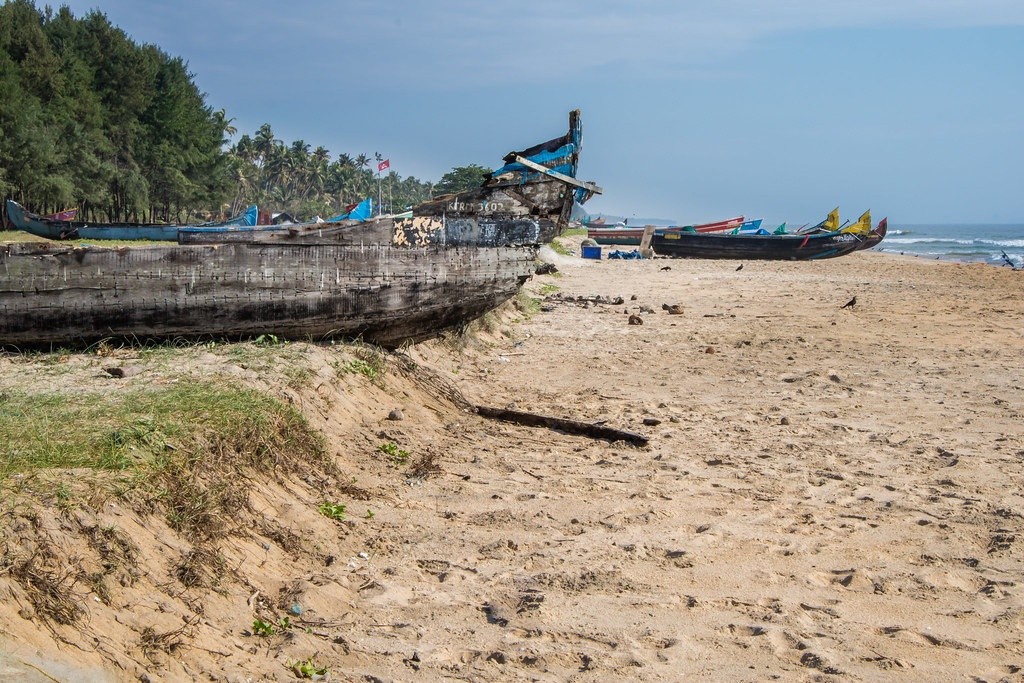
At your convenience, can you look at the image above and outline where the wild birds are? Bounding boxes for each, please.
[735,263,744,271]
[840,295,857,310]
[660,266,672,272]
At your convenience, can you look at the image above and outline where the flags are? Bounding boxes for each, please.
[378,159,390,171]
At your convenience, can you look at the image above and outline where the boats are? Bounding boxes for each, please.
[0,106,605,354]
[5,196,260,241]
[580,205,888,251]
[176,193,374,245]
[649,209,872,262]
